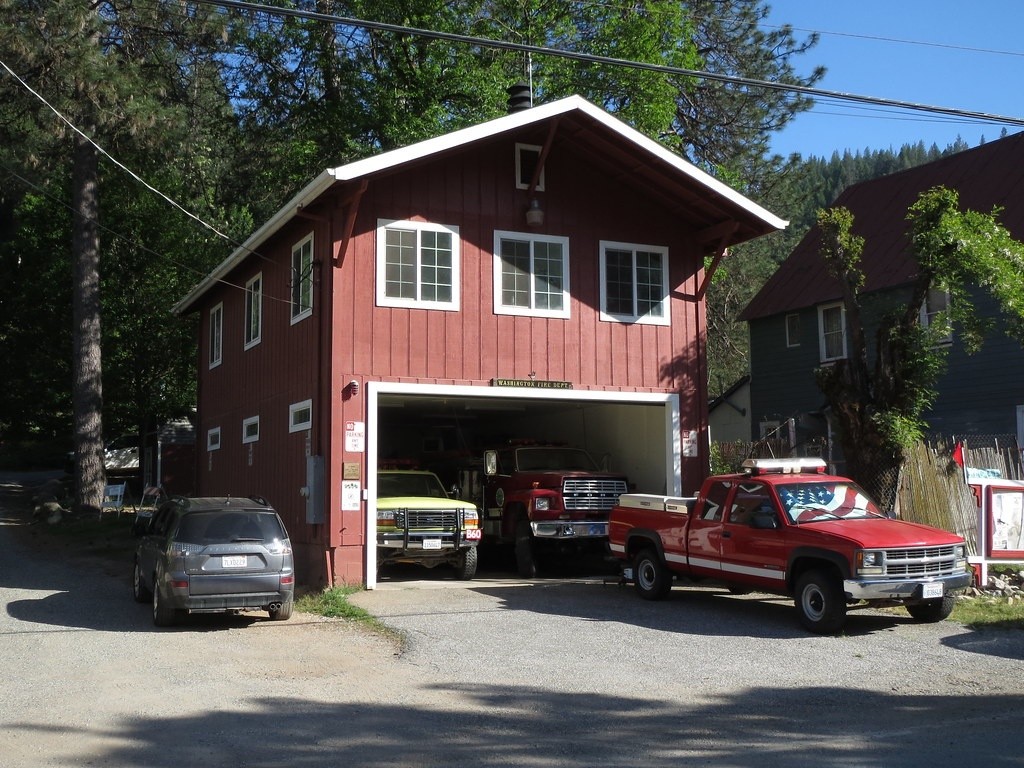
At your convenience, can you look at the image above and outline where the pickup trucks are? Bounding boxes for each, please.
[607,457,973,635]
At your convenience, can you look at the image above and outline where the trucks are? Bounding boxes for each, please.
[377,456,486,582]
[456,437,636,579]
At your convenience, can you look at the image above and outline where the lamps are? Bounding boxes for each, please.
[527,198,545,226]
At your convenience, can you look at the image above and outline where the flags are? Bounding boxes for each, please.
[777,482,878,523]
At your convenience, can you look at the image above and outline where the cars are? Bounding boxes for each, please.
[63,431,142,484]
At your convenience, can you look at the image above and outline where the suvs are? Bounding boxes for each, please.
[132,493,296,628]
